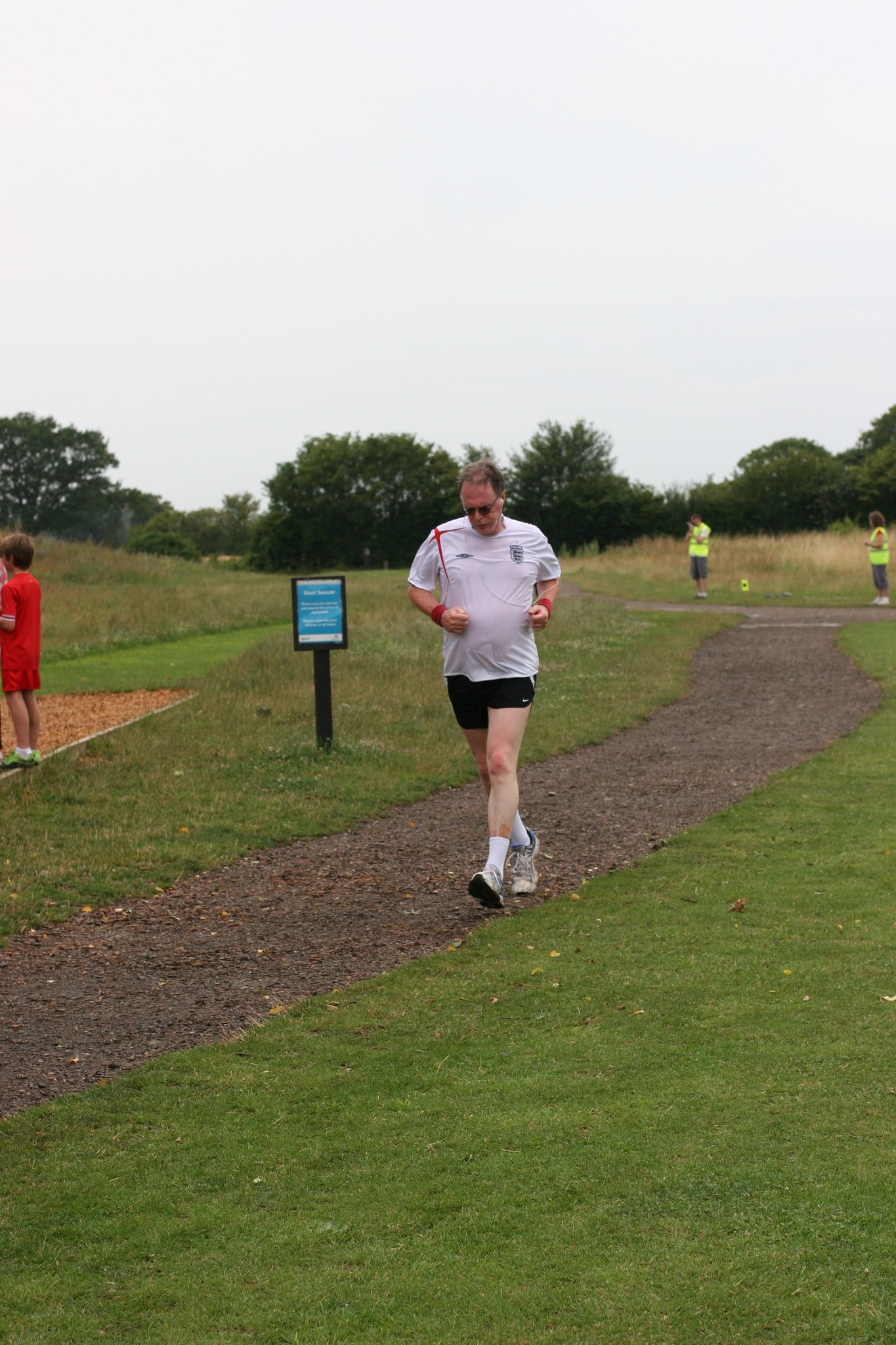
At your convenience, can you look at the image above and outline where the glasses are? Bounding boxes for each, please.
[461,496,498,516]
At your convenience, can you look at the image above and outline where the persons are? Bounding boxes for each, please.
[865,511,890,606]
[407,462,563,907]
[686,515,712,599]
[0,535,41,769]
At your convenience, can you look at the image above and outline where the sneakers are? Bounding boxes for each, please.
[32,749,41,766]
[509,826,540,894]
[0,750,36,769]
[468,863,506,910]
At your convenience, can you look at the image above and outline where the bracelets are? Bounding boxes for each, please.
[431,605,449,627]
[538,598,553,619]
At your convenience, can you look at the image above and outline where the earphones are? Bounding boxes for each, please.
[500,498,503,506]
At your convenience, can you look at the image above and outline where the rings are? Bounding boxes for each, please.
[536,624,538,628]
